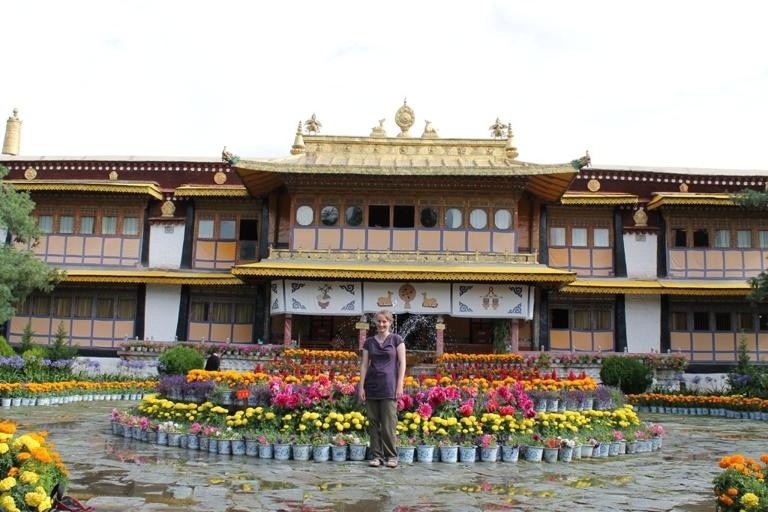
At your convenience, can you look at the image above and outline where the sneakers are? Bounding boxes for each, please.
[368,459,381,467]
[386,457,398,468]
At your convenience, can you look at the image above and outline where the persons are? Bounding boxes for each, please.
[204,348,220,371]
[358,311,407,467]
